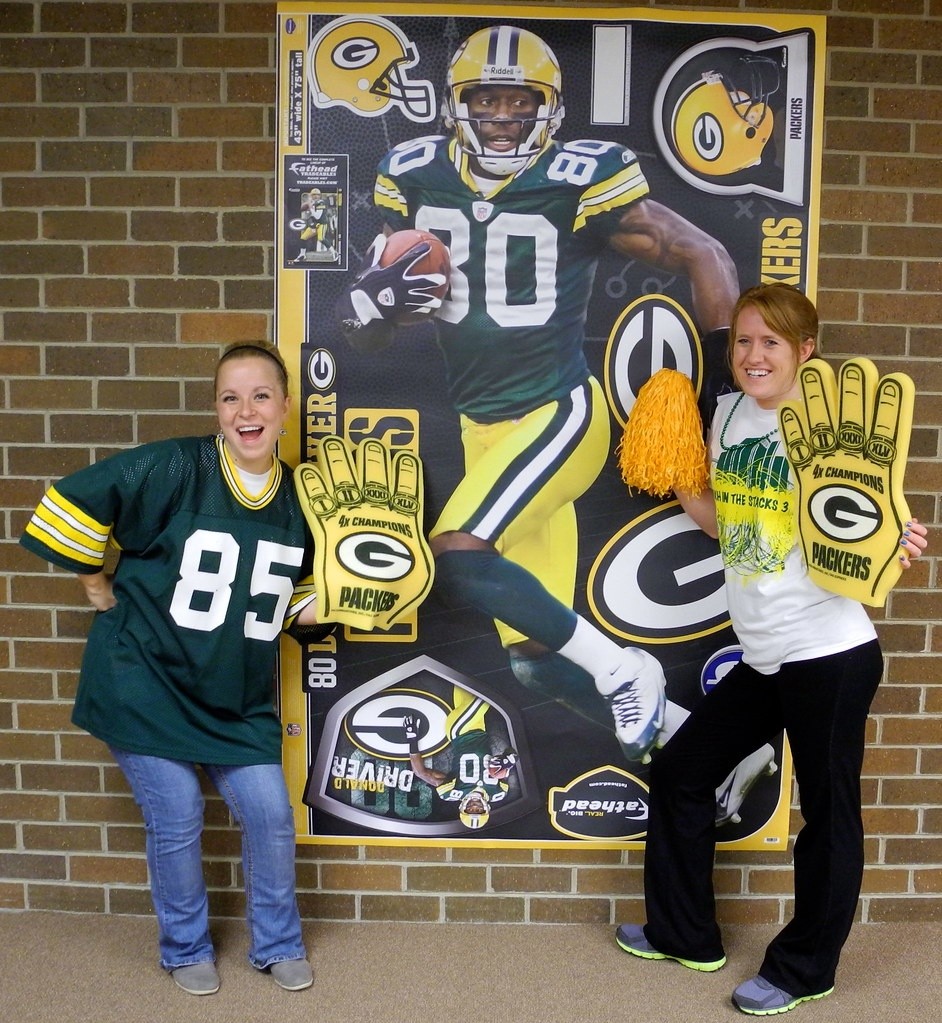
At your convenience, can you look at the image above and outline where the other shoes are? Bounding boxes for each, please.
[171,958,220,994]
[269,957,314,991]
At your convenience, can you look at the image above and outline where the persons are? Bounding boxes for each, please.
[292,187,340,263]
[611,282,931,1015]
[317,23,779,831]
[14,338,348,999]
[400,713,519,829]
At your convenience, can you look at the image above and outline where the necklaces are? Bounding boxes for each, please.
[719,392,778,450]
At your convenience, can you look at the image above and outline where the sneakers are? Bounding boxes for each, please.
[731,976,834,1017]
[616,923,726,971]
[714,743,778,829]
[595,647,666,765]
[333,252,338,262]
[293,255,306,263]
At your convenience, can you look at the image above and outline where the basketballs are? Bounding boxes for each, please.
[378,226,451,321]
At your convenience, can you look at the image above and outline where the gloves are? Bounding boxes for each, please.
[402,715,422,753]
[488,749,518,779]
[333,234,446,331]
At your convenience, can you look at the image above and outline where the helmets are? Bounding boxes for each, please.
[446,26,562,174]
[310,188,321,195]
[458,795,488,828]
[671,56,781,175]
[311,20,432,118]
